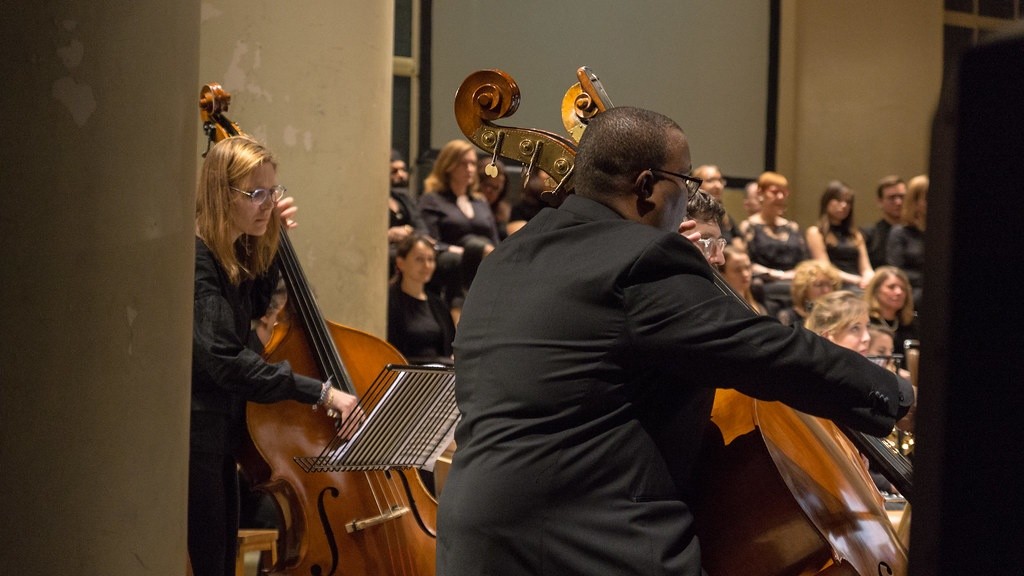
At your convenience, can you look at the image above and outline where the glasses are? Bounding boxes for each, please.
[769,188,789,196]
[705,176,727,185]
[232,184,287,204]
[698,237,728,259]
[648,167,704,201]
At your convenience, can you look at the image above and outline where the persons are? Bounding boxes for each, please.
[387,139,929,498]
[906,33,1024,576]
[436,107,918,576]
[187,135,367,576]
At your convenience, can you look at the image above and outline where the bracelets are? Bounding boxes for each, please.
[317,374,332,405]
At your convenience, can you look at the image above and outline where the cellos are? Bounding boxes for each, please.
[200,81,440,576]
[453,66,914,576]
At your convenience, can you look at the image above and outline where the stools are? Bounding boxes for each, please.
[235,529,278,576]
